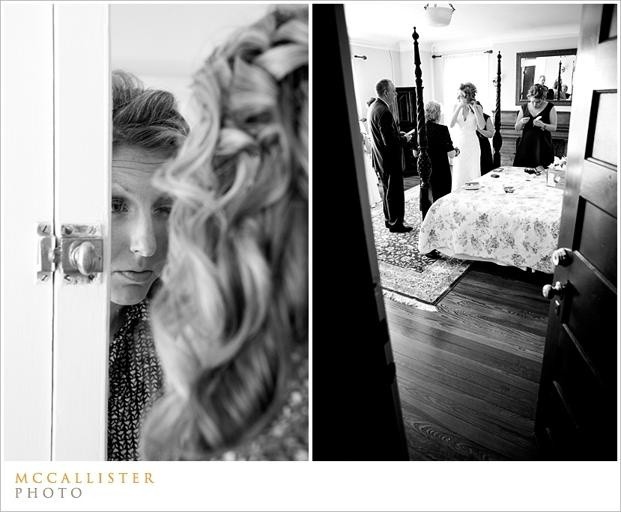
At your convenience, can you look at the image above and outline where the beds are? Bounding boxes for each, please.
[409,26,566,273]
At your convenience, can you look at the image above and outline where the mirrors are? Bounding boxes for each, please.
[516,48,577,108]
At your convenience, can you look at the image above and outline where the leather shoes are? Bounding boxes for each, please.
[385,220,414,233]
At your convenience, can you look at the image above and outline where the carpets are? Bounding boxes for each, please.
[367,177,485,307]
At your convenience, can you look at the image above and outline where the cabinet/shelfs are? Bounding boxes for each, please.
[393,86,424,178]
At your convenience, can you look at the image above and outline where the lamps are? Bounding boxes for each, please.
[423,3,457,27]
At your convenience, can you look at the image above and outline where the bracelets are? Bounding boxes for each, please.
[540,124,546,131]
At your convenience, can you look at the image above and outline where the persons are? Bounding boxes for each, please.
[424,101,460,203]
[451,83,486,192]
[108,70,189,461]
[367,79,413,233]
[515,86,557,169]
[536,75,569,99]
[471,101,494,176]
[138,4,308,461]
